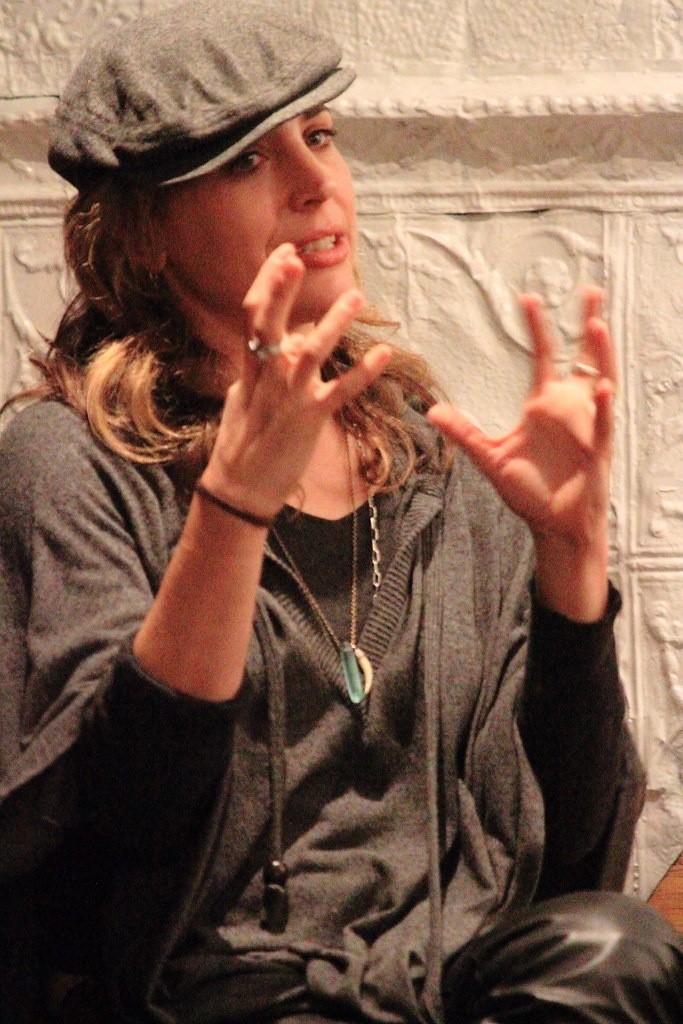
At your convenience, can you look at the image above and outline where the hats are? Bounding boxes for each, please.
[48,0,357,194]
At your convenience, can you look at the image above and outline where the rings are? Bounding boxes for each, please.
[570,360,600,378]
[247,338,288,364]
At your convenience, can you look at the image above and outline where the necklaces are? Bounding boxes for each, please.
[274,418,374,708]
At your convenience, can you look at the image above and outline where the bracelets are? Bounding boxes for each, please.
[193,481,275,531]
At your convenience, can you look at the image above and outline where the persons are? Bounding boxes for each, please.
[0,1,683,1024]
